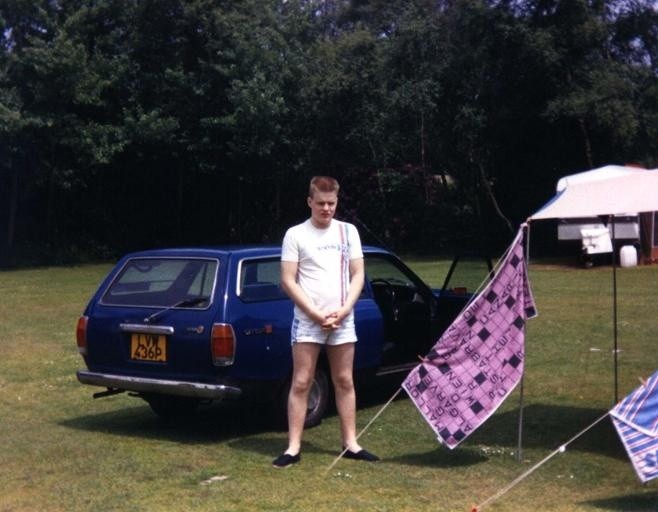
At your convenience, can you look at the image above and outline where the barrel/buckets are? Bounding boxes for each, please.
[619,244,637,268]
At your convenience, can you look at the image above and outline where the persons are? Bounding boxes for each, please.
[271,176,379,467]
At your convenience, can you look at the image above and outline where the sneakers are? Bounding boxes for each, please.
[272,452,301,469]
[342,445,379,463]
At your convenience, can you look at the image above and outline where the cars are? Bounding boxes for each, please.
[75,243,496,430]
